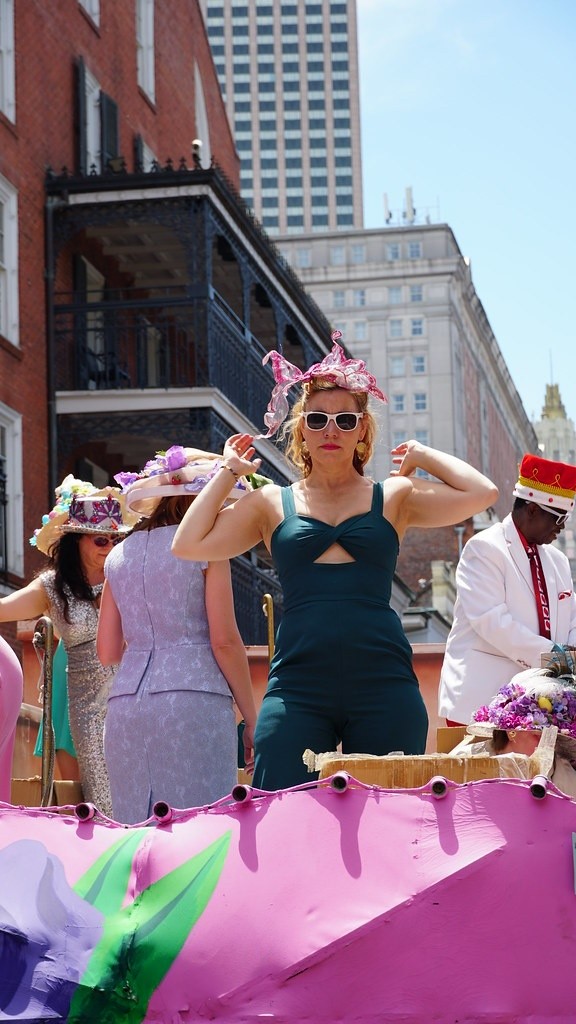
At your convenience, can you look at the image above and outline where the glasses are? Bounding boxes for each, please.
[298,411,364,434]
[526,500,570,526]
[91,535,124,548]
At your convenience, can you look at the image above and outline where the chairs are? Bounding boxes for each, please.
[72,338,134,391]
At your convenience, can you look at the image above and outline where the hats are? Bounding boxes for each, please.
[466,643,576,740]
[512,453,576,513]
[53,495,134,535]
[112,446,271,519]
[30,496,71,560]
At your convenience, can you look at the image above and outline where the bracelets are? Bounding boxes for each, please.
[221,466,239,485]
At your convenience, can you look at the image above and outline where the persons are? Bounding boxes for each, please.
[97,446,257,825]
[171,329,499,797]
[438,455,576,728]
[0,474,136,823]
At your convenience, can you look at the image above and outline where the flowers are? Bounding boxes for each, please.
[472,682,576,738]
[29,445,247,547]
